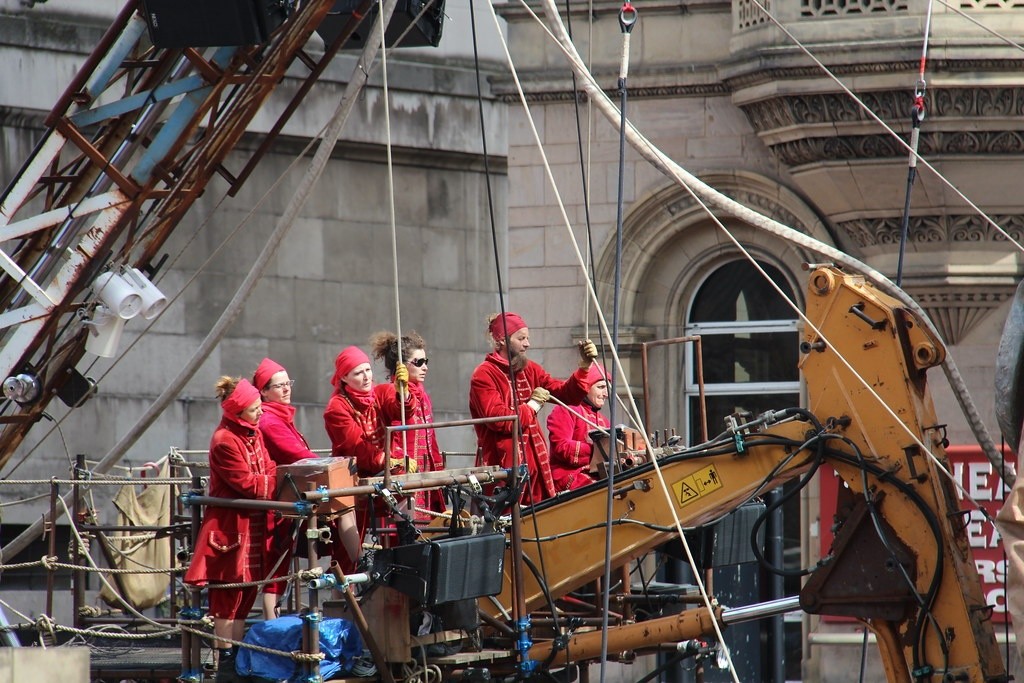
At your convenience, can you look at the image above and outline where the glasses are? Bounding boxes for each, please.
[403,358,429,367]
[264,379,295,391]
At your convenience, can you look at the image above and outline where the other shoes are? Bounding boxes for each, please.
[215,660,256,683]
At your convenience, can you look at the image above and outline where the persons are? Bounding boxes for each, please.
[323,345,418,575]
[546,361,611,492]
[468,312,598,517]
[371,328,447,545]
[184,374,278,683]
[251,358,320,622]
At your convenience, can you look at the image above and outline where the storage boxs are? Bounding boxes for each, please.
[276,457,360,516]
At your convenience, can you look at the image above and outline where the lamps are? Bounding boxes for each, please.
[84,265,167,357]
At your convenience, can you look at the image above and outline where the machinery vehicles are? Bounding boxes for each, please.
[2,0,1013,683]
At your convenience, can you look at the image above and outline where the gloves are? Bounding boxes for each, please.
[389,456,419,474]
[527,387,551,415]
[393,362,409,393]
[578,338,598,370]
